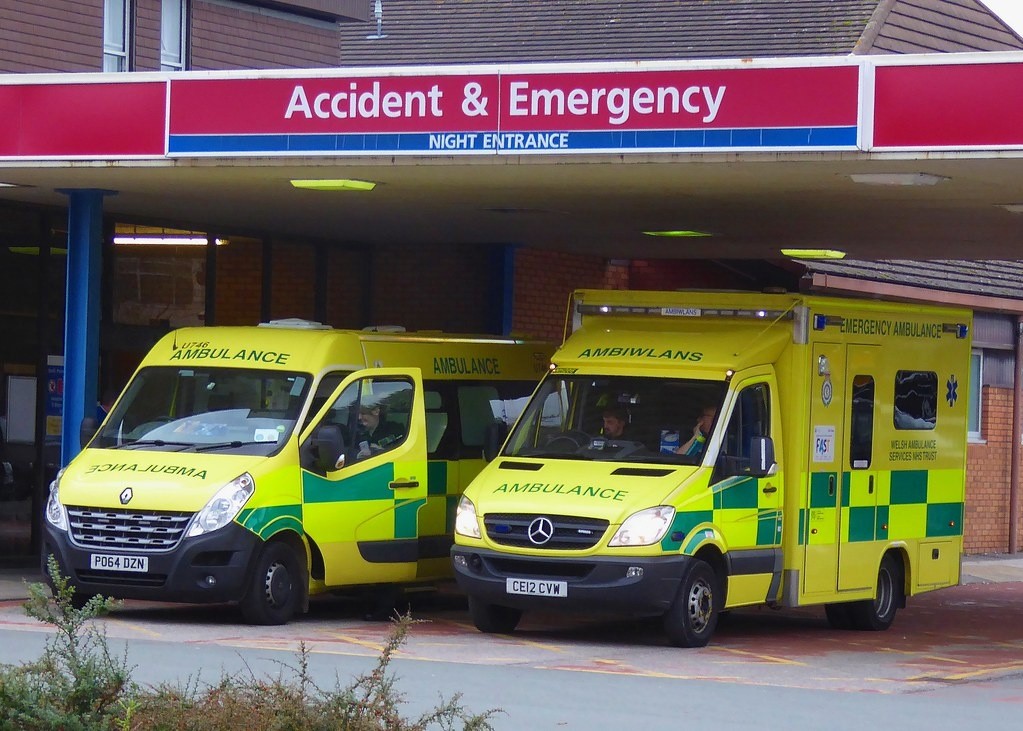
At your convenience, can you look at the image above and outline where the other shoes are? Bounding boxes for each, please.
[363,607,400,621]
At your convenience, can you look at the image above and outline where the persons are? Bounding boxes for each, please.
[674,405,728,456]
[343,395,409,619]
[97,387,126,444]
[602,406,654,450]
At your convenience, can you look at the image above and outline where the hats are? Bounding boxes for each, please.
[359,395,387,414]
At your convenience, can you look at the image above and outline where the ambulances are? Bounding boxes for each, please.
[39,317,556,625]
[449,288,974,648]
